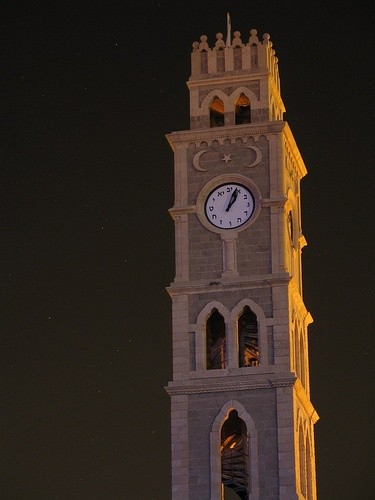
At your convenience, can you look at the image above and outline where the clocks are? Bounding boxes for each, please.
[204,181,256,231]
